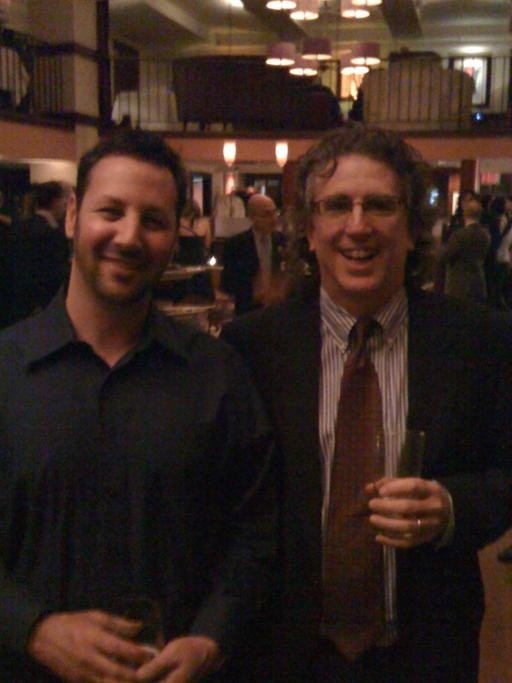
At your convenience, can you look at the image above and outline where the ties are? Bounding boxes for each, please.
[321,316,388,662]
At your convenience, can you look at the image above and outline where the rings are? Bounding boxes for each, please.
[416,517,423,532]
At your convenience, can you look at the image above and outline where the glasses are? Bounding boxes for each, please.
[311,196,405,217]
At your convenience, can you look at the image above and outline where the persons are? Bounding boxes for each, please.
[214,125,512,681]
[1,178,512,327]
[1,128,280,681]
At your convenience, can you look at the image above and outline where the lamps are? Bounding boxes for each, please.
[263,0,381,78]
[223,137,236,167]
[275,139,289,167]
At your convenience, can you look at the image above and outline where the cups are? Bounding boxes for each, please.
[117,613,167,682]
[375,433,423,538]
